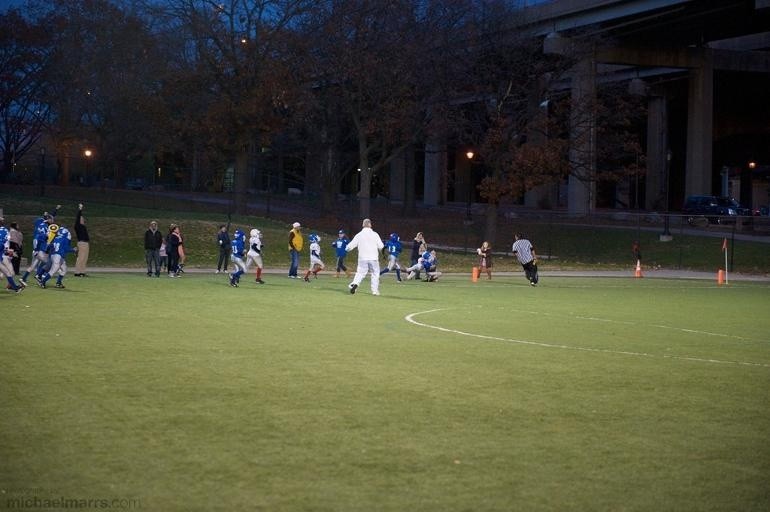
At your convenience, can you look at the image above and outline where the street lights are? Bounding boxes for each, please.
[37,147,48,196]
[463,148,477,224]
[83,149,93,187]
[660,148,673,242]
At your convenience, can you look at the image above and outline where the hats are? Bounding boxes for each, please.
[292,222,301,229]
[338,229,345,234]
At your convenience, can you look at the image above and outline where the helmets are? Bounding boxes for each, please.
[250,228,261,238]
[308,233,318,243]
[44,214,71,240]
[422,251,432,259]
[389,232,401,242]
[0,217,7,227]
[233,228,245,240]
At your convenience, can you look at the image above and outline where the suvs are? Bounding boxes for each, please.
[682,192,753,227]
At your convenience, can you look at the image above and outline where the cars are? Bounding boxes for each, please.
[753,205,770,235]
[226,186,349,201]
[66,174,149,191]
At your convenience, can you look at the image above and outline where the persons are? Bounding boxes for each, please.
[145,221,185,278]
[477,241,493,280]
[214,219,443,297]
[513,232,539,285]
[0,203,90,293]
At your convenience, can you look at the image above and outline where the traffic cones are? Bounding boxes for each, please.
[633,258,645,278]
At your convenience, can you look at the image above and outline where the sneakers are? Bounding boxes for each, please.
[5,273,89,294]
[288,272,317,282]
[215,269,264,287]
[350,284,357,294]
[146,270,181,278]
